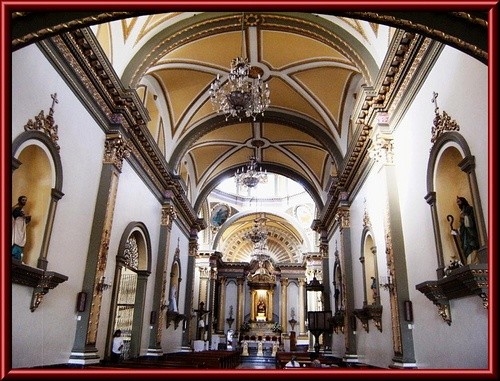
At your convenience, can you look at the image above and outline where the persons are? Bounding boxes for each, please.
[285,356,300,368]
[111,329,124,359]
[450,196,480,265]
[309,355,321,368]
[12,195,32,259]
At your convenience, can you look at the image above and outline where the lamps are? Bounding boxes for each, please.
[209,12,271,122]
[234,156,269,189]
